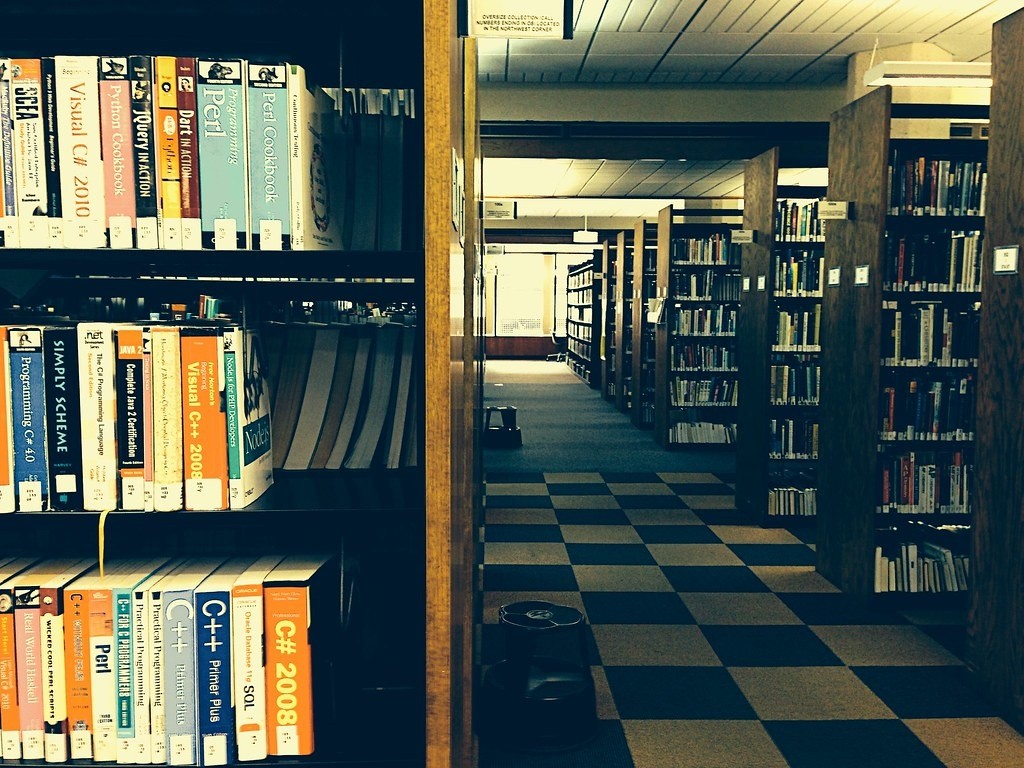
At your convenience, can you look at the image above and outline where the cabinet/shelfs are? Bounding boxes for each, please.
[0,0,487,768]
[601,238,619,405]
[735,144,830,528]
[631,219,655,431]
[615,230,636,413]
[565,249,602,389]
[816,85,989,609]
[965,5,1024,734]
[656,203,745,451]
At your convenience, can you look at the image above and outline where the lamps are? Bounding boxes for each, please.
[864,39,993,89]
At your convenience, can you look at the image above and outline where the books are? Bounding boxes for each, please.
[568,157,987,594]
[0,56,418,768]
